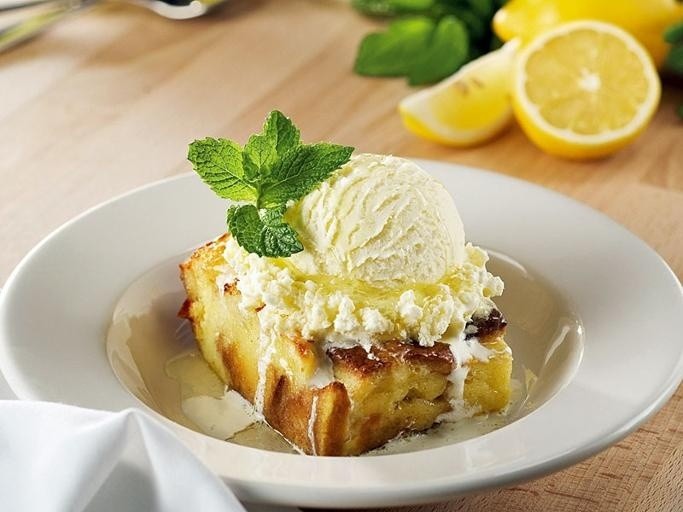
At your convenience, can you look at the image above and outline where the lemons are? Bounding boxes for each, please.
[489,0,682,69]
[510,20,663,160]
[399,39,521,147]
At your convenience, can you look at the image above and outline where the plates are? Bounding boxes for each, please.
[4,154,683,506]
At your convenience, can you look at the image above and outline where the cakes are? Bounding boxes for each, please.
[177,109,513,456]
